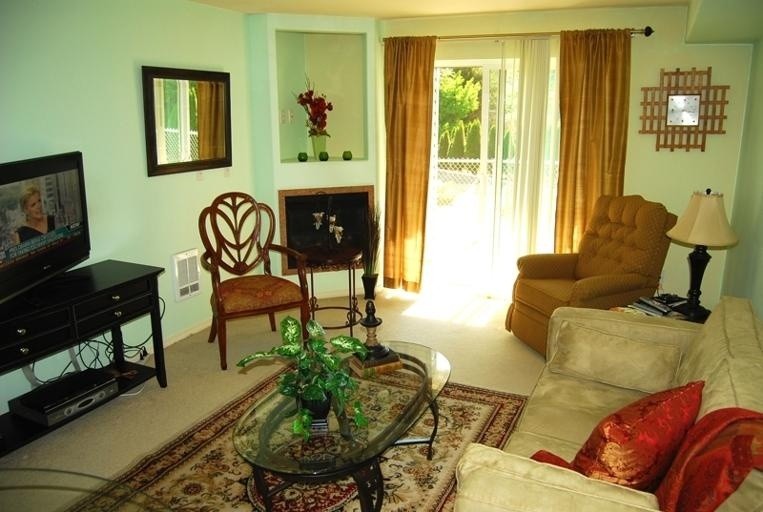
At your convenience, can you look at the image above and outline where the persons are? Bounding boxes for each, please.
[14,186,59,247]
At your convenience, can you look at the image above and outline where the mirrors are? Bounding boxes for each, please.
[141,65,234,179]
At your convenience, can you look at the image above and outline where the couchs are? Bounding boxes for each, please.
[454,297,763,512]
[505,194,678,360]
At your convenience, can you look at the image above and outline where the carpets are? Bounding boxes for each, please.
[55,354,529,512]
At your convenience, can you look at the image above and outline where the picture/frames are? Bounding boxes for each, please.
[278,184,375,277]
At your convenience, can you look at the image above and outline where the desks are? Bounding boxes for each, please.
[0,259,168,458]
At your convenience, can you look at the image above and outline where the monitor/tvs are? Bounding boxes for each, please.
[0,151,91,305]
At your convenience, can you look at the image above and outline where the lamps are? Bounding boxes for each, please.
[665,188,739,324]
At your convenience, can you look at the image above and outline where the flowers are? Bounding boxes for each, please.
[291,72,334,135]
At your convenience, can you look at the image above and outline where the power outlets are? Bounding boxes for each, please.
[135,350,153,365]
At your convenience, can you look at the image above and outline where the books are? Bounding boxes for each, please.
[348,345,403,378]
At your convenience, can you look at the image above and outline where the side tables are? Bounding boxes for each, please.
[608,293,712,324]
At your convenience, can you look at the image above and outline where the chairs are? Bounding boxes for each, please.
[198,193,311,370]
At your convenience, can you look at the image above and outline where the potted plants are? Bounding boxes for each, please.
[237,316,374,444]
[360,201,380,300]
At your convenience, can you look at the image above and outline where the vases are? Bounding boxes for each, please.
[310,136,327,159]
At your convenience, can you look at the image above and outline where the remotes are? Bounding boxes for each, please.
[640,296,671,313]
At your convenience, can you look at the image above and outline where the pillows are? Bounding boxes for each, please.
[571,379,708,488]
